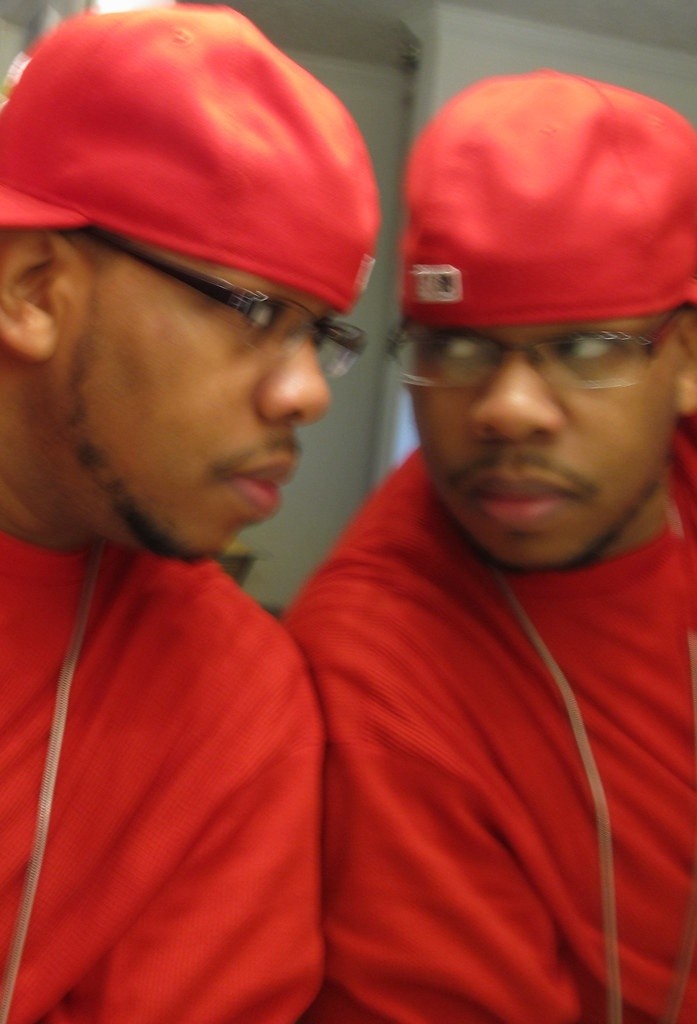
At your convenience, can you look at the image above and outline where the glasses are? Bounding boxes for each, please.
[383,304,685,388]
[62,220,366,377]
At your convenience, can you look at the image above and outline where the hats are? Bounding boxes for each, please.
[1,5,380,309]
[400,69,697,324]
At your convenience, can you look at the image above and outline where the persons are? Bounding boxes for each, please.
[2,0,380,1024]
[281,68,696,1022]
